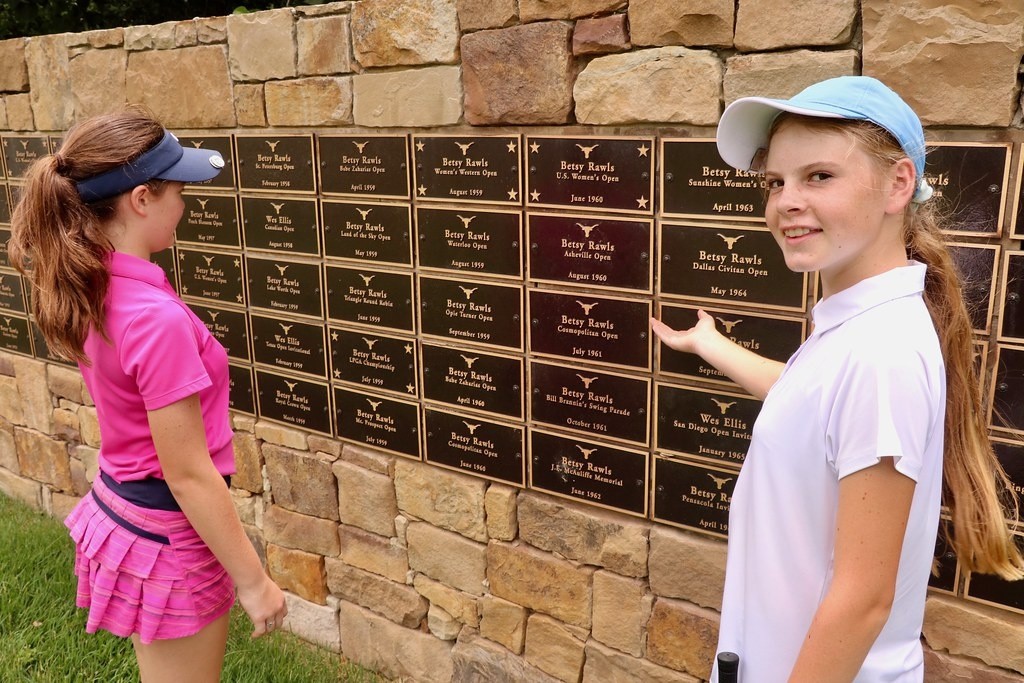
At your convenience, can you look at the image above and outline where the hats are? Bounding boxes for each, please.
[716,76,933,214]
[73,123,223,203]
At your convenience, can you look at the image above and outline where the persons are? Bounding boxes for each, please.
[647,75,1024,683]
[7,112,289,683]
